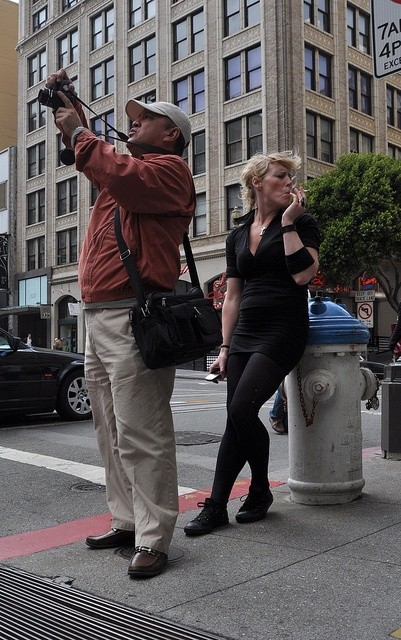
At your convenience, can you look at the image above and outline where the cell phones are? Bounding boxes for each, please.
[205,370,220,380]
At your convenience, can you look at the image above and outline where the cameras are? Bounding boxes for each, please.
[39,81,74,109]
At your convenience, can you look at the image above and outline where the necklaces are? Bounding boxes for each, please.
[254,216,272,236]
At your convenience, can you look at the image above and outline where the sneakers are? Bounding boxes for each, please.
[269,417,284,434]
[184,498,229,534]
[236,485,273,523]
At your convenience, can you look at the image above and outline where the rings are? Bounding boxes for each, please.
[299,196,305,207]
[305,202,308,208]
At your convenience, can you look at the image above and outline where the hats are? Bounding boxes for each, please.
[125,98,191,147]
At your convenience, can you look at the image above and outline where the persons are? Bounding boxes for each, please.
[38,64,198,580]
[184,146,323,536]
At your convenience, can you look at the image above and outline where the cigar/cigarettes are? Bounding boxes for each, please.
[295,189,309,193]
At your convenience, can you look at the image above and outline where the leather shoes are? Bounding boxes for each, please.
[86,528,135,548]
[128,546,168,575]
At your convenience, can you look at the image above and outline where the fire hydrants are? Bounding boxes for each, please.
[279,290,382,505]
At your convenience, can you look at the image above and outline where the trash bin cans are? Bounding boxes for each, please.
[382,363,401,460]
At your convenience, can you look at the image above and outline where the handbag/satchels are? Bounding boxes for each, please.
[129,286,223,369]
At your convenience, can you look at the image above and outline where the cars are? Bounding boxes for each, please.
[0,327,92,421]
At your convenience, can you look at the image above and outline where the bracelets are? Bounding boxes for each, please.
[281,224,298,236]
[220,344,229,349]
